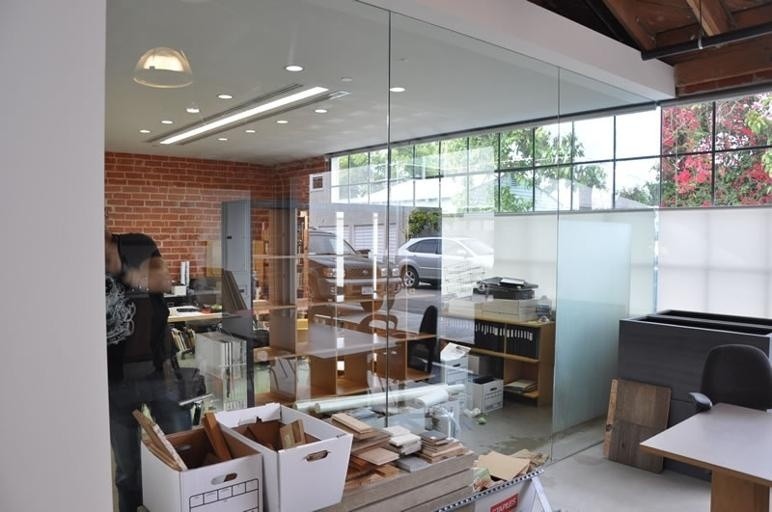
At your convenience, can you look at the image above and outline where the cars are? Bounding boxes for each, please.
[396,237,493,295]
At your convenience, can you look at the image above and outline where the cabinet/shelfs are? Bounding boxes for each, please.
[221,200,441,411]
[439,312,554,408]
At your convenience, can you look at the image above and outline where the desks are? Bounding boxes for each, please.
[638,401,772,512]
[166,301,270,323]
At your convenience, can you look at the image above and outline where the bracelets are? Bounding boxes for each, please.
[110,260,127,280]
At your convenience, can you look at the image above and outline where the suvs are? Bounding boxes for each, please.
[299,222,400,315]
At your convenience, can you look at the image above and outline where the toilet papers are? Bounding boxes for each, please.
[415,390,450,409]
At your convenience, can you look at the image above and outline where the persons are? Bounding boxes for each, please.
[105,225,194,511]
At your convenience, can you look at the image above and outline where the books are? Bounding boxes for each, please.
[503,378,536,392]
[172,328,196,351]
[415,428,550,482]
[204,266,248,314]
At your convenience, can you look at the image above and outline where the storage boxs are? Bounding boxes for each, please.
[457,377,504,413]
[215,402,353,512]
[443,358,468,390]
[476,473,538,512]
[140,430,264,512]
